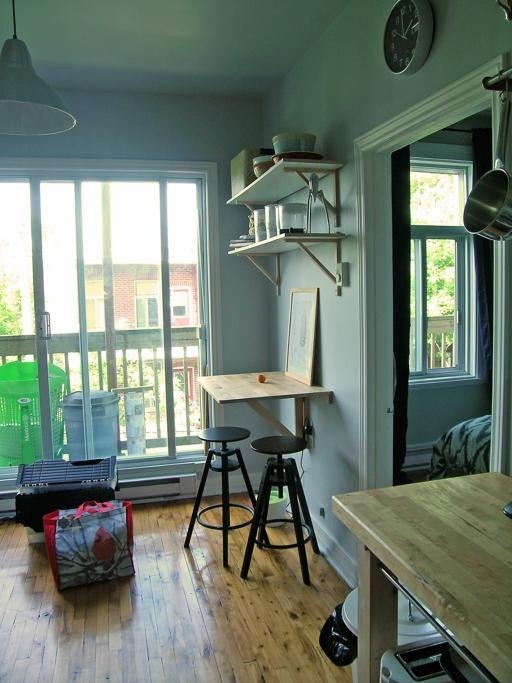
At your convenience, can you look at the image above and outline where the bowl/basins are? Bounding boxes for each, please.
[270,132,317,153]
[252,156,274,179]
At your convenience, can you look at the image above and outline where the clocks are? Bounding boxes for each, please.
[382,0,434,77]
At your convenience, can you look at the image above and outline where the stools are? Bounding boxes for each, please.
[179,425,323,588]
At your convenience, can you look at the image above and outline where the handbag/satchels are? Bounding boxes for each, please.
[43,498,137,592]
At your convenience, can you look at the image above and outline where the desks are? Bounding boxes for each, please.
[329,468,511,683]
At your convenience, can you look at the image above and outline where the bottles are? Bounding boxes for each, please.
[253,203,308,243]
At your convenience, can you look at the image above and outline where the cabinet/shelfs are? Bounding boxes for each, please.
[226,157,349,297]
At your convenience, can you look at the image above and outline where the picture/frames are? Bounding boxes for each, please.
[284,288,321,386]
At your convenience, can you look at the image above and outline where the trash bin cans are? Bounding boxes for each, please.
[318,581,454,682]
[57,390,120,460]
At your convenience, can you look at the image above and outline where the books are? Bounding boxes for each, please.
[228,243,254,248]
[229,238,255,243]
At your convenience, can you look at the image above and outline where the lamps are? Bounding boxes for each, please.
[1,0,78,136]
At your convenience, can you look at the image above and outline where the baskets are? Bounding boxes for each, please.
[0,360,67,466]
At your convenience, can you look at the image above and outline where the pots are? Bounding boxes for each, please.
[463,91,512,240]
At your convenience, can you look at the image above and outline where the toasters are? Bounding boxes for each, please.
[378,634,464,683]
